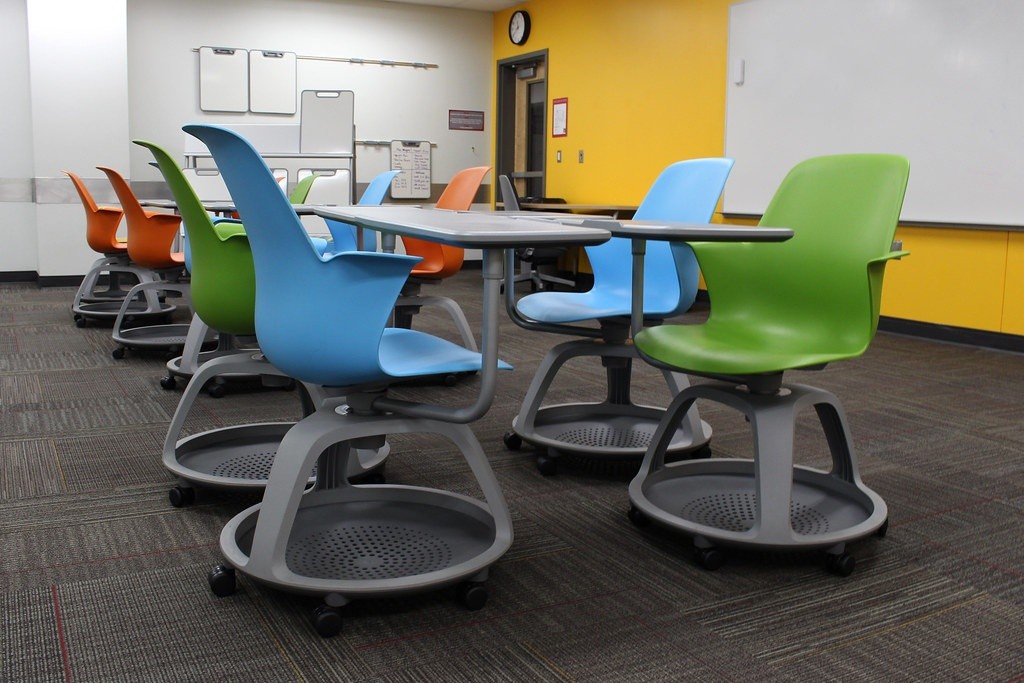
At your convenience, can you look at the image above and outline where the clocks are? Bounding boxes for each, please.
[508,10,531,46]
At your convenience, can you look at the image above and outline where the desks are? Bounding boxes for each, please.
[312,203,612,428]
[458,210,794,344]
[129,198,337,226]
[520,203,643,291]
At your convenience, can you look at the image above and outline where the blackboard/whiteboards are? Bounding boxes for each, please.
[299,90,354,156]
[721,0,1024,231]
[248,49,297,116]
[267,168,289,198]
[179,168,233,236]
[297,168,351,237]
[198,46,249,114]
[390,139,432,200]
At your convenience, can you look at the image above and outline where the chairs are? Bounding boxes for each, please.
[231,177,285,219]
[499,174,576,295]
[132,140,392,512]
[393,166,493,330]
[503,157,737,478]
[181,123,517,639]
[627,153,912,579]
[284,174,321,205]
[324,171,401,257]
[62,170,181,330]
[147,161,300,398]
[96,166,243,362]
